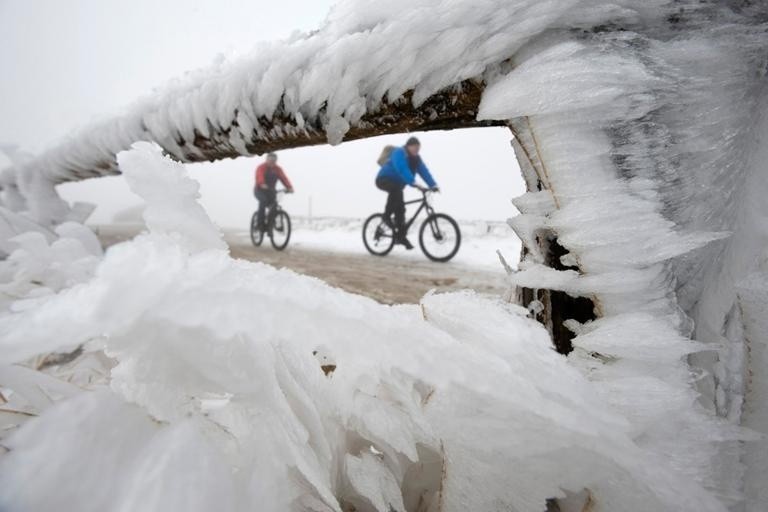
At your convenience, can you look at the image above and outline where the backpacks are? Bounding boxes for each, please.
[377,145,407,167]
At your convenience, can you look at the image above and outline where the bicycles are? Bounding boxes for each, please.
[361,180,462,263]
[249,184,293,253]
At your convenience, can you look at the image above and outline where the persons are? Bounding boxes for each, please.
[378,134,442,249]
[255,151,294,234]
